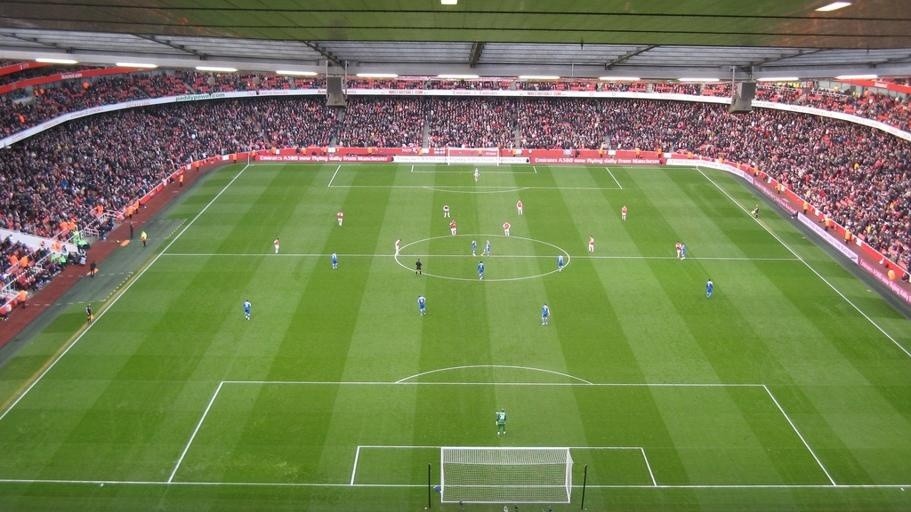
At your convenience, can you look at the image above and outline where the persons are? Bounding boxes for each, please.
[679,241,686,261]
[336,209,345,227]
[475,168,481,183]
[273,236,282,255]
[620,205,630,222]
[417,295,428,317]
[706,277,715,299]
[442,203,451,218]
[733,164,911,304]
[394,239,402,257]
[0,161,202,332]
[470,240,479,257]
[587,234,596,254]
[331,251,339,270]
[481,239,493,257]
[415,258,424,278]
[495,409,507,437]
[476,260,486,283]
[449,219,459,237]
[540,305,552,327]
[502,221,512,238]
[676,240,683,259]
[555,252,565,273]
[517,199,524,216]
[242,299,253,321]
